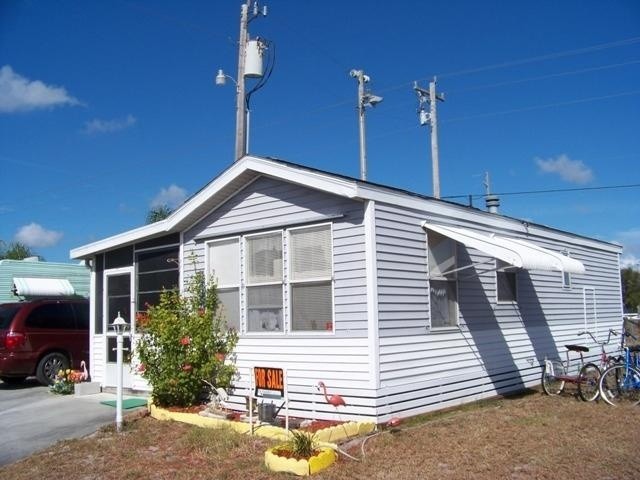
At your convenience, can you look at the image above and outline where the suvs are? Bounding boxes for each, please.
[1,300,90,390]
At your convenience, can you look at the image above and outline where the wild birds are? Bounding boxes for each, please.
[80,360,89,381]
[316,381,350,444]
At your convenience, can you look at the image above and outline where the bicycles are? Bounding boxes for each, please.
[542,329,640,408]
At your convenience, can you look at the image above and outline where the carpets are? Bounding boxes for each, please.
[98,397,148,411]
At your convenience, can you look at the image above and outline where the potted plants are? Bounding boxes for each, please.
[263,426,337,477]
